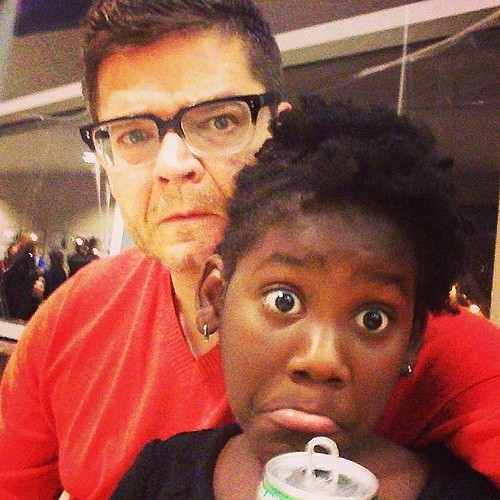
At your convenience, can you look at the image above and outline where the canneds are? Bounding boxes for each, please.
[253,436,380,499]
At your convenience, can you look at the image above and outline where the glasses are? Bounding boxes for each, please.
[78,90,279,174]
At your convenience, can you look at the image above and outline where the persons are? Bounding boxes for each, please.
[107,90,500,500]
[0,3,500,500]
[0,224,106,319]
[449,275,486,318]
